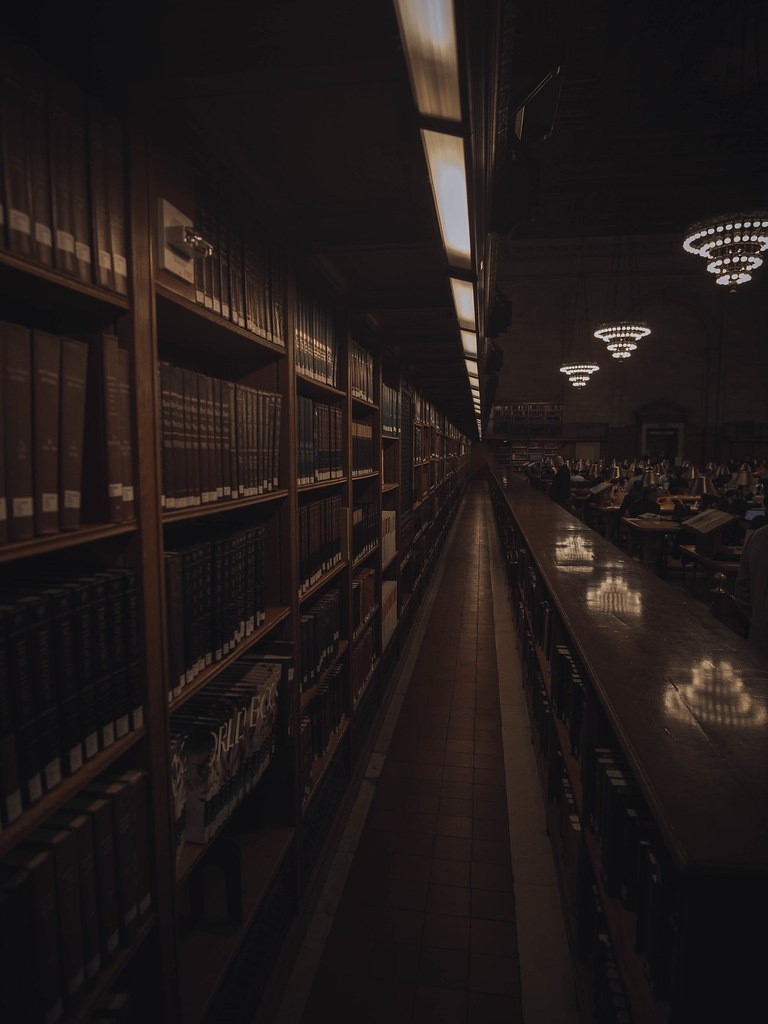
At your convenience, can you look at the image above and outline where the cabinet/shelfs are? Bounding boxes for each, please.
[492,401,565,470]
[0,42,470,1024]
[488,471,768,1024]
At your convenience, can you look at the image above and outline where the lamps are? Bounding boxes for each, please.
[559,181,601,391]
[593,196,652,365]
[682,0,768,296]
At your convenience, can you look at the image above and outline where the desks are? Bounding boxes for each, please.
[619,517,682,569]
[590,503,699,540]
[678,544,742,602]
[570,493,701,522]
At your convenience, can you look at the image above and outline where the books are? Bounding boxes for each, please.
[0,315,135,542]
[0,757,157,1024]
[153,121,286,347]
[158,516,266,705]
[680,508,734,533]
[296,490,349,808]
[491,403,566,474]
[352,329,467,711]
[295,280,345,487]
[0,24,133,300]
[156,361,283,510]
[488,471,685,1024]
[170,643,298,932]
[0,561,145,832]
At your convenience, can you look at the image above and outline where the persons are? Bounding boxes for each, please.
[734,524,768,653]
[535,449,768,560]
